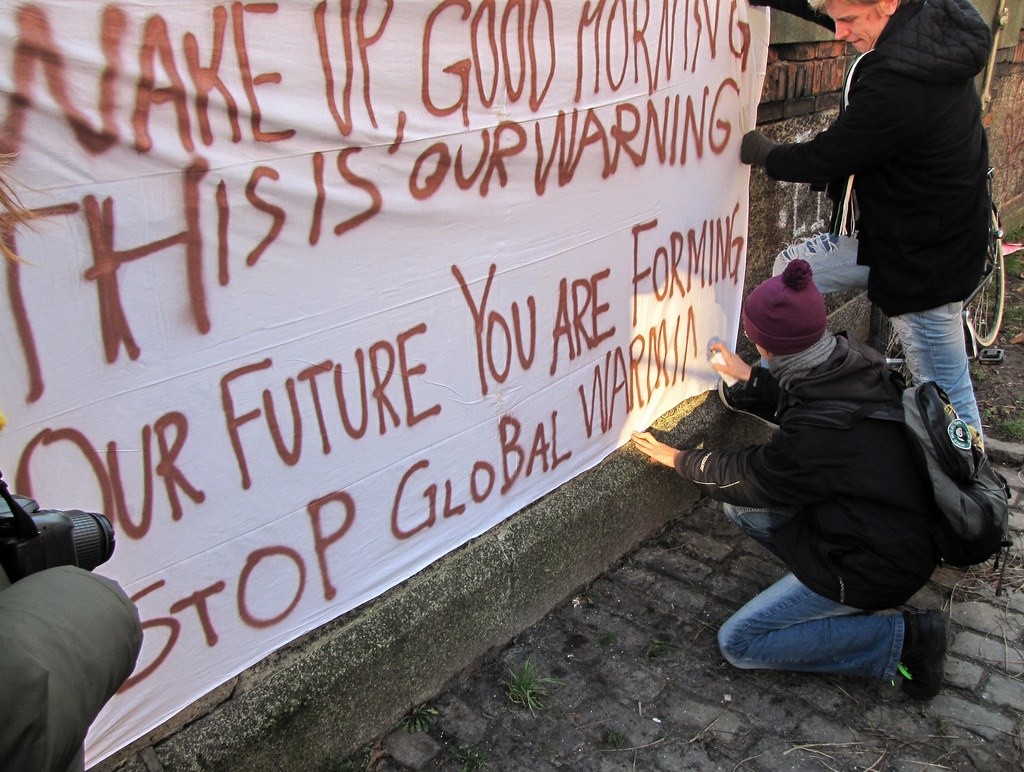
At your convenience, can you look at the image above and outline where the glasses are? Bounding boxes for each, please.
[745,332,754,343]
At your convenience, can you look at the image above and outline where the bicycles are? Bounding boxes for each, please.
[870,158,1005,389]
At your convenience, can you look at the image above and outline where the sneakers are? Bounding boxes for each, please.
[718,380,780,432]
[903,606,949,704]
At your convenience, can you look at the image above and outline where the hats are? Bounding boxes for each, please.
[743,258,826,354]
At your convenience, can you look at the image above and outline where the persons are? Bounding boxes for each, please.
[742,0,994,467]
[631,258,946,700]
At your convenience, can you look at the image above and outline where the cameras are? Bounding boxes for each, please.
[0,494,116,584]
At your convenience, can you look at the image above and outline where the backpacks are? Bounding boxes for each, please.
[778,368,1014,595]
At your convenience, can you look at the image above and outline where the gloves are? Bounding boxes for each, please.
[740,130,774,175]
